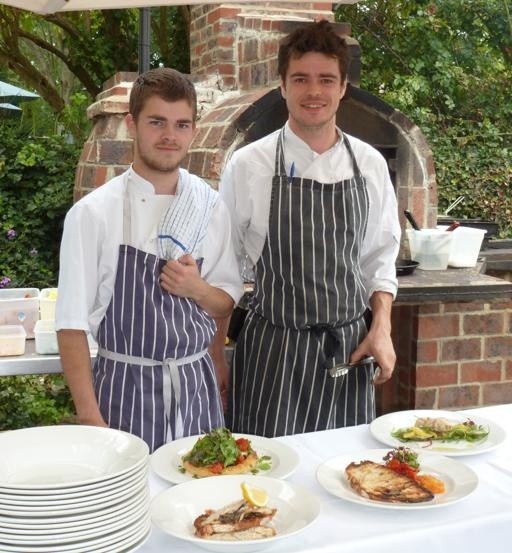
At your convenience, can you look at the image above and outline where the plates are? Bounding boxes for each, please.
[369,409,505,456]
[153,474,320,547]
[1,425,149,552]
[151,431,304,484]
[315,449,479,511]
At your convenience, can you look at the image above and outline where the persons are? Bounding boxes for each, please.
[206,21,402,439]
[54,68,244,454]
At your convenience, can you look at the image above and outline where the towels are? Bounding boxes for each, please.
[158,167,220,260]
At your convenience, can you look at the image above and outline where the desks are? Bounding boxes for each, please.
[0,336,101,376]
